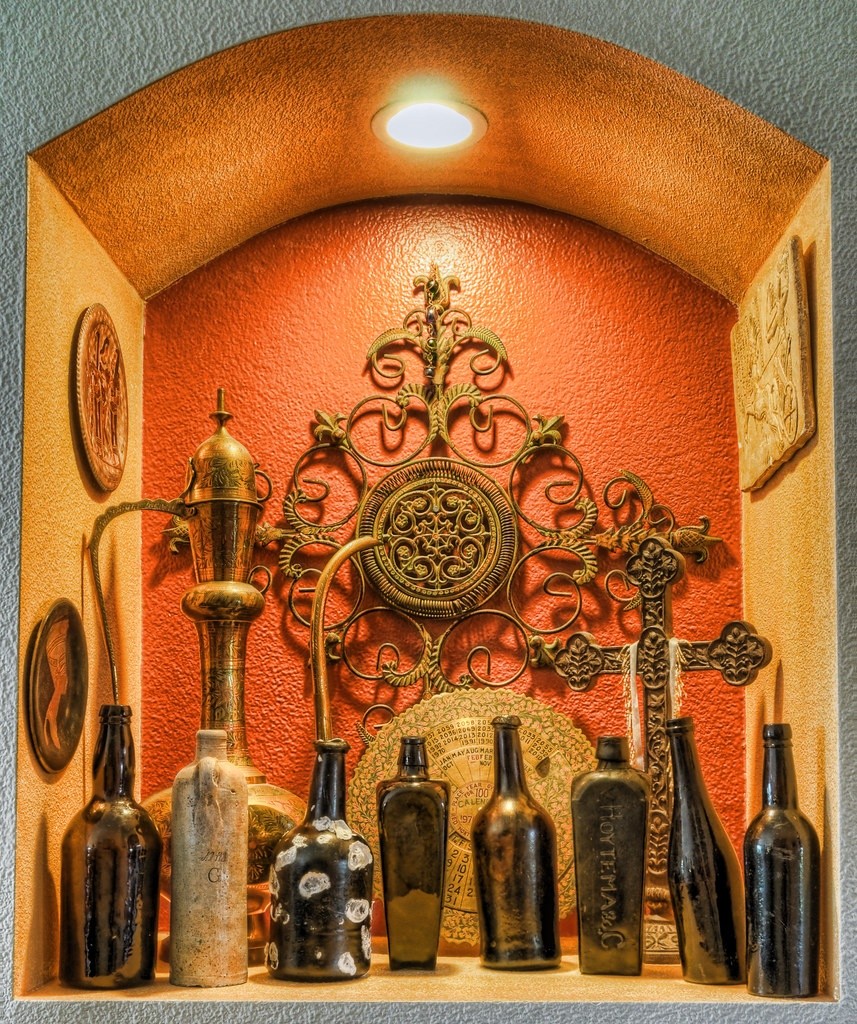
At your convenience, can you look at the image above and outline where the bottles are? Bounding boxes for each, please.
[373,734,452,970]
[741,724,823,1001]
[661,716,751,985]
[265,740,374,978]
[57,706,157,991]
[168,727,254,985]
[569,733,649,975]
[469,714,562,970]
